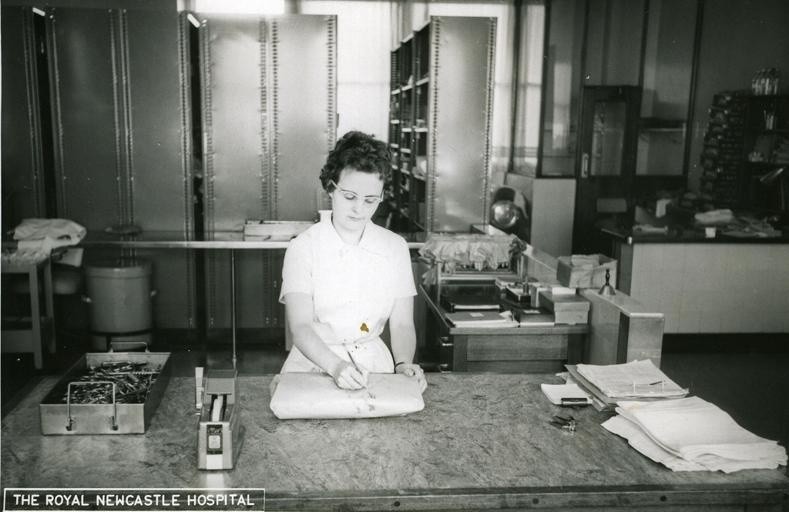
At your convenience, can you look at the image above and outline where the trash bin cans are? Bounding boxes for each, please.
[81,258,158,353]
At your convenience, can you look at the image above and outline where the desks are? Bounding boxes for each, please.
[594,219,789,335]
[4,371,788,511]
[69,229,289,371]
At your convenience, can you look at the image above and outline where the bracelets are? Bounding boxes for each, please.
[394,360,405,367]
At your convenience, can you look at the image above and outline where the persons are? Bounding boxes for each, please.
[274,130,429,395]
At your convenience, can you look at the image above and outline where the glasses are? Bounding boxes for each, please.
[328,180,385,206]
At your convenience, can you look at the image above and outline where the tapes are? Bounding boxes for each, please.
[210,395,224,422]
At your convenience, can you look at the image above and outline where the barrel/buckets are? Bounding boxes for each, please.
[81,258,156,335]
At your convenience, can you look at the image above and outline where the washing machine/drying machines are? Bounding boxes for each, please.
[634,198,673,226]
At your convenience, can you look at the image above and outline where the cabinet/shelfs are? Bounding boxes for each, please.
[743,95,789,216]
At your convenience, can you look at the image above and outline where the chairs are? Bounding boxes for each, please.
[596,197,629,222]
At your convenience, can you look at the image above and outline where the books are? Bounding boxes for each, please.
[444,310,554,328]
[557,357,690,414]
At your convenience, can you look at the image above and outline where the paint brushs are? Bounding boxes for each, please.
[343,342,372,399]
